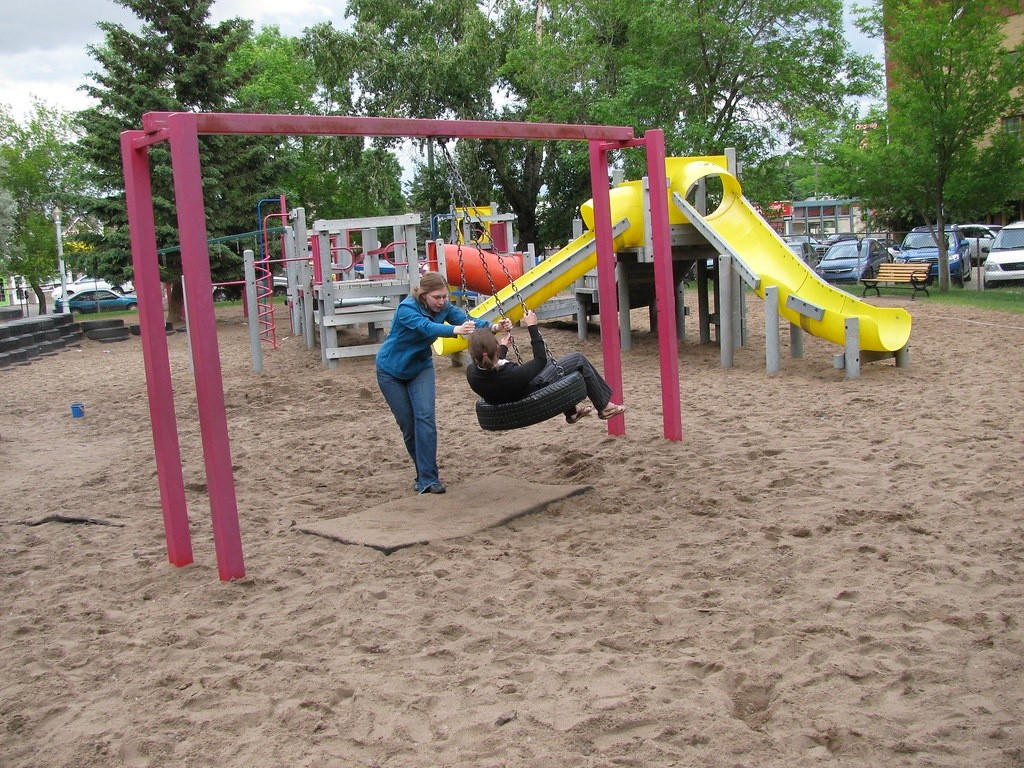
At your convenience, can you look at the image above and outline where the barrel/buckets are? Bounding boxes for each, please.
[71,403,84,418]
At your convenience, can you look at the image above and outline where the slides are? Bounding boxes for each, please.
[671,189,914,353]
[432,229,626,357]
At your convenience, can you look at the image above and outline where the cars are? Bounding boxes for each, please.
[957,224,1003,267]
[864,238,902,259]
[208,268,288,303]
[815,239,895,284]
[50,274,135,301]
[783,232,860,272]
[984,220,1024,289]
[52,288,138,316]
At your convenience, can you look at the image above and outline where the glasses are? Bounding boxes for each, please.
[427,293,449,300]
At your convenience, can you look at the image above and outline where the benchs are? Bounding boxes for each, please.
[860,263,933,301]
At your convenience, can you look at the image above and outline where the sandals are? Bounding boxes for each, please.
[601,404,626,419]
[566,404,594,424]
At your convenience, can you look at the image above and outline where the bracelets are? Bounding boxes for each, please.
[494,324,499,332]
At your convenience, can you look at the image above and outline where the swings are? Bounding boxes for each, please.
[436,136,588,432]
[217,237,243,298]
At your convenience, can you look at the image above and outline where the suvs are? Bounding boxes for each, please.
[893,223,972,288]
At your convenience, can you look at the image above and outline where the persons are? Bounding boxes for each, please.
[466,310,626,424]
[376,272,513,493]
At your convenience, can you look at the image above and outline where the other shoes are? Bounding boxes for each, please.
[414,483,419,490]
[426,484,446,494]
